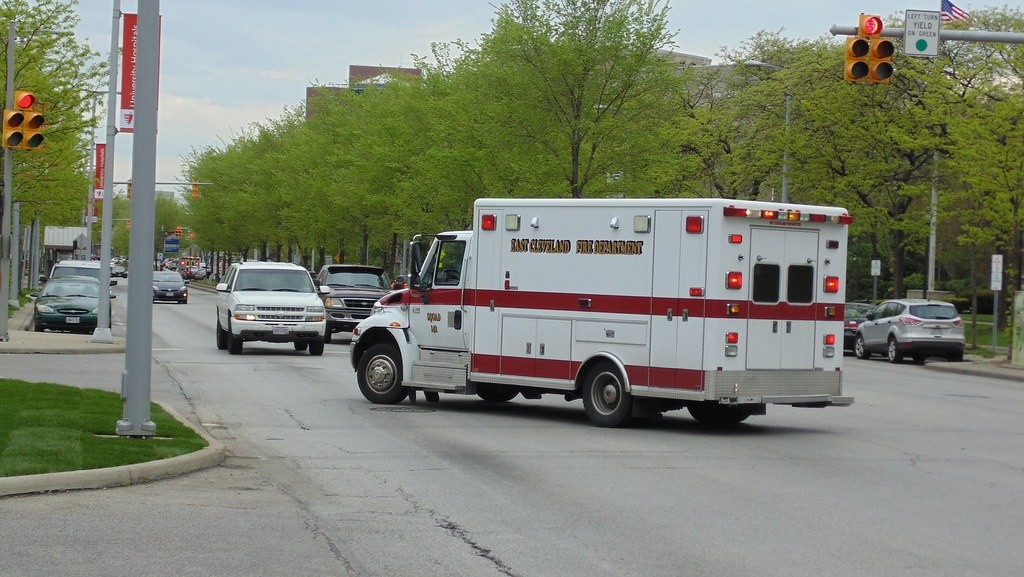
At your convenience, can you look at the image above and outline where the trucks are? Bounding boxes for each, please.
[349,196,855,428]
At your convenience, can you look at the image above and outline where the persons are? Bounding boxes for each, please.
[391,276,407,290]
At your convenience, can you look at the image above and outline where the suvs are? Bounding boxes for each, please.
[214,260,326,356]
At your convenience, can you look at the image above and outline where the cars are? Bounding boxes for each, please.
[315,264,391,343]
[30,275,117,334]
[47,259,118,293]
[843,303,878,349]
[110,255,228,281]
[853,298,966,364]
[152,270,190,305]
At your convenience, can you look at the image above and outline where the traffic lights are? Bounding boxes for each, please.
[1,90,46,148]
[175,226,182,237]
[842,13,894,85]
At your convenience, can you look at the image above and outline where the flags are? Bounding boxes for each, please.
[940,0,972,23]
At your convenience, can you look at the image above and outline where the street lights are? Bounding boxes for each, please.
[745,59,793,203]
